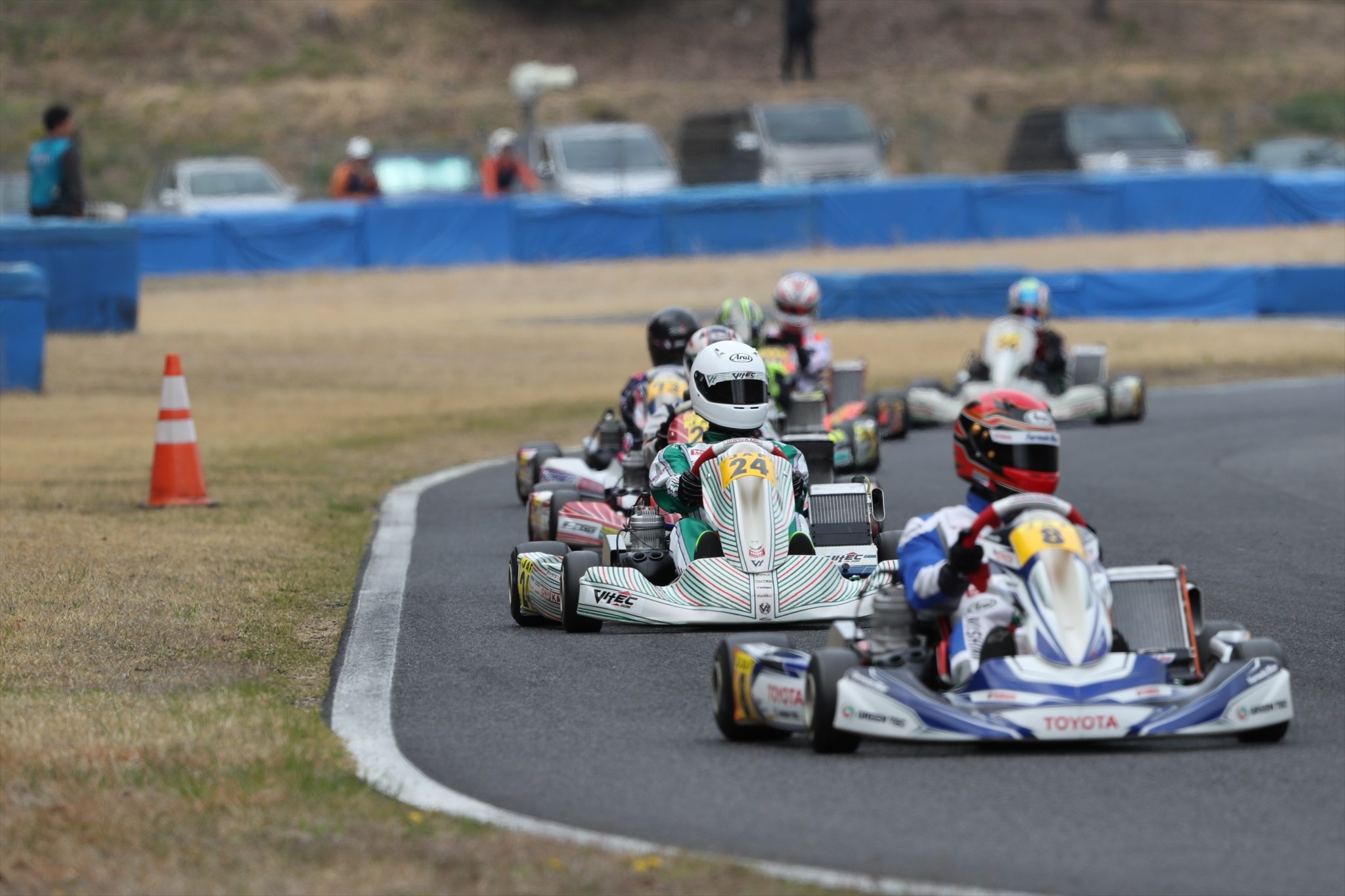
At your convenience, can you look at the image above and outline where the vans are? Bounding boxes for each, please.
[671,96,899,191]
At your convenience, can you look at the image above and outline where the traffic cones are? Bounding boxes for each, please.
[141,352,220,508]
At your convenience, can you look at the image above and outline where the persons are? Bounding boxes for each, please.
[779,0,817,82]
[900,384,1130,690]
[25,103,84,218]
[479,127,548,201]
[585,273,834,574]
[331,137,382,199]
[967,277,1068,397]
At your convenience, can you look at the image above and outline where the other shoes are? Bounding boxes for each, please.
[1109,628,1130,653]
[694,530,724,558]
[788,531,816,556]
[980,624,1015,661]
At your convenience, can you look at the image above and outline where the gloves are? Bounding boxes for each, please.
[792,469,804,498]
[949,526,985,576]
[676,470,702,503]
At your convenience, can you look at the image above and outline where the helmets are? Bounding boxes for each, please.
[1006,277,1048,312]
[714,296,763,346]
[684,325,746,374]
[771,272,818,333]
[647,308,700,366]
[755,343,801,400]
[667,409,710,445]
[953,389,1061,495]
[688,340,770,430]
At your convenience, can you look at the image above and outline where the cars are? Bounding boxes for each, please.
[512,117,681,199]
[139,153,305,214]
[999,102,1220,180]
